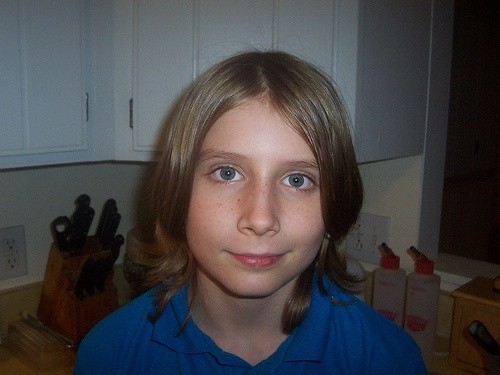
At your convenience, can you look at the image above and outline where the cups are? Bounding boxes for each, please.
[487,353,500,375]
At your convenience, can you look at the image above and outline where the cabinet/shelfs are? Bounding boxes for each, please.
[0,0,109,171]
[273,0,432,165]
[108,0,273,163]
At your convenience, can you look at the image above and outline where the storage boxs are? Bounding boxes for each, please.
[36,233,118,351]
[448,275,500,375]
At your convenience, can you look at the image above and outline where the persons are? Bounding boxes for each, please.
[73,48,426,375]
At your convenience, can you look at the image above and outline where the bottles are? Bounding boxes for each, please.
[370,241,407,330]
[405,245,441,364]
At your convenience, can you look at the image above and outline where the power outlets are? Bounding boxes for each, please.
[344,212,391,265]
[0,225,28,281]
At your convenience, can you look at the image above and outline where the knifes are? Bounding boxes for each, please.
[20,311,74,349]
[51,194,125,301]
